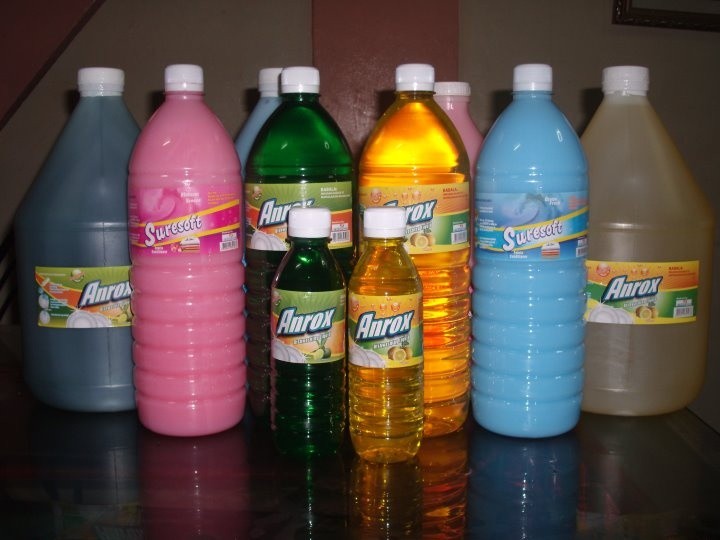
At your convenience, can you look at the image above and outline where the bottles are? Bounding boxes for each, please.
[15,64,712,438]
[30,408,690,538]
[271,207,350,456]
[346,208,424,464]
